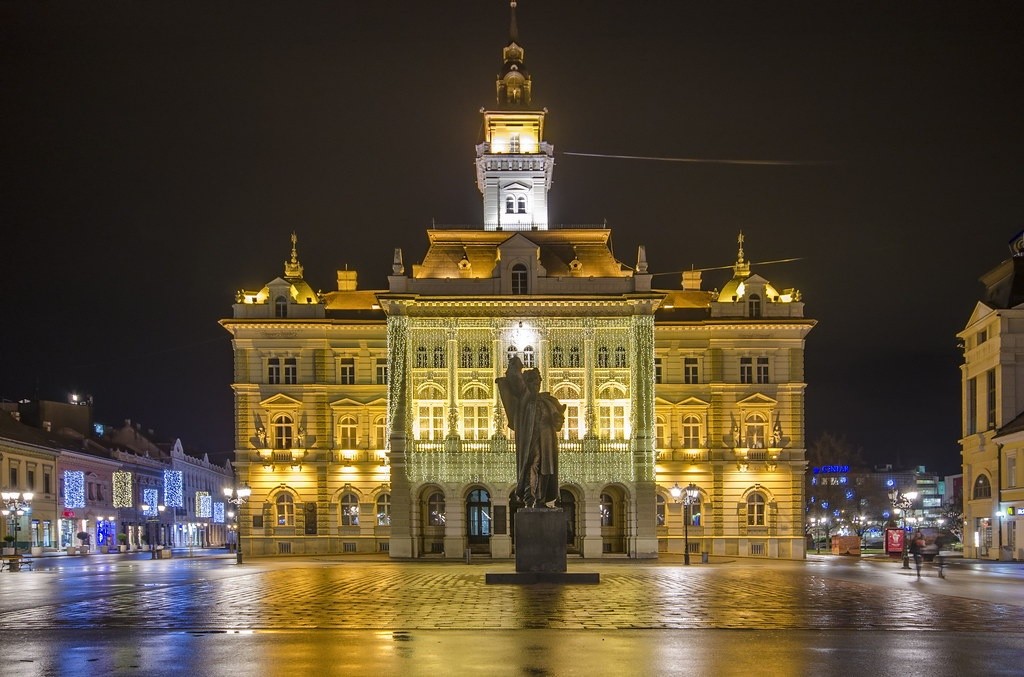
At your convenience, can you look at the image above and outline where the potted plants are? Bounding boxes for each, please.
[77,532,90,556]
[118,532,128,553]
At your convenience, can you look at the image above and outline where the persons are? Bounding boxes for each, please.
[298,426,306,449]
[257,427,266,448]
[733,425,741,448]
[494,352,568,509]
[773,425,781,447]
[911,531,926,582]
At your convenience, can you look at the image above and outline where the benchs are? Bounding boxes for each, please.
[0,554,33,572]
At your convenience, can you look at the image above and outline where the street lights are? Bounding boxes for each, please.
[198,522,209,549]
[889,489,917,569]
[669,483,699,565]
[142,502,168,559]
[98,513,115,547]
[2,488,35,570]
[224,482,252,564]
[228,522,238,553]
